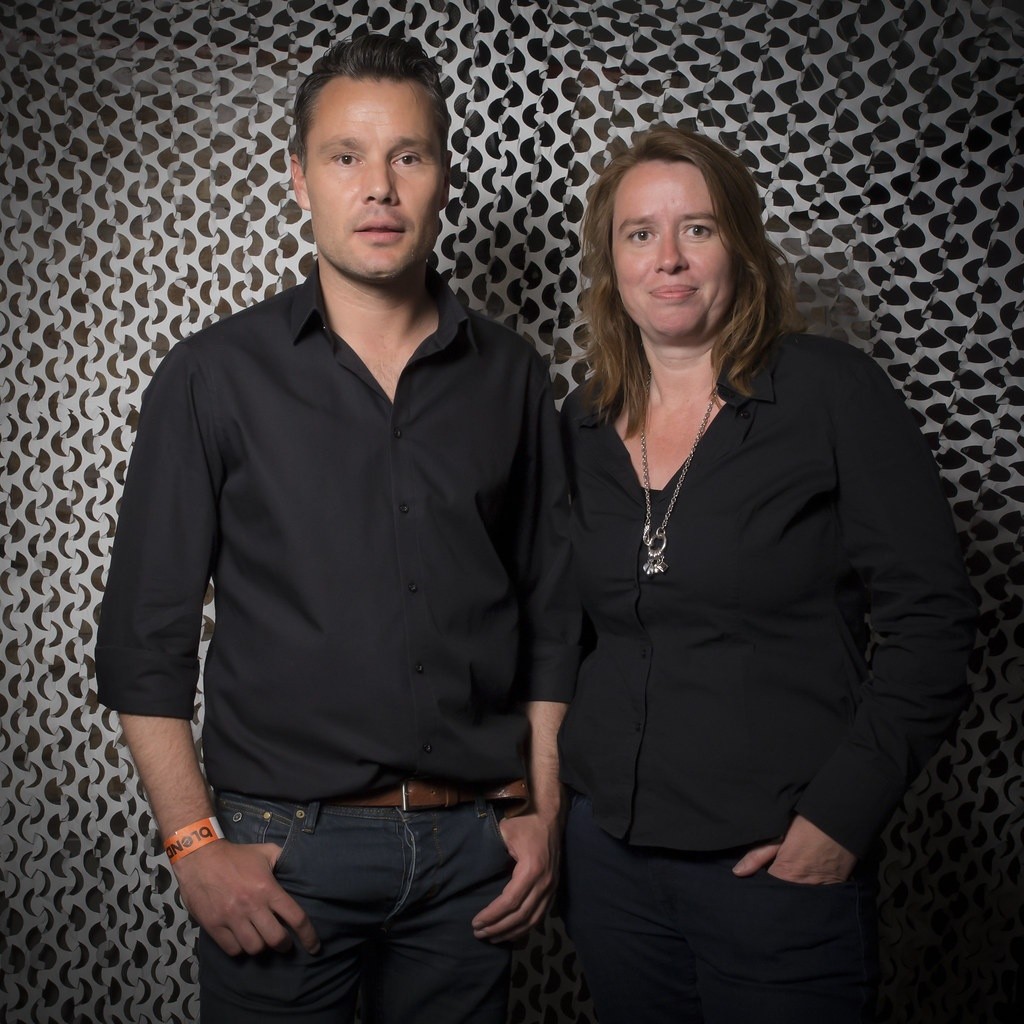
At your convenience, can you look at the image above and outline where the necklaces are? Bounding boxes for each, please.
[634,356,718,576]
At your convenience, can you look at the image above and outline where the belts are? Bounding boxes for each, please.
[329,777,531,820]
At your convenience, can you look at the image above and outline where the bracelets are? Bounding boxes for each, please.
[160,813,229,867]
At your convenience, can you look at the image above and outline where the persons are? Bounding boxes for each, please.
[91,27,582,1024]
[556,115,982,1024]
